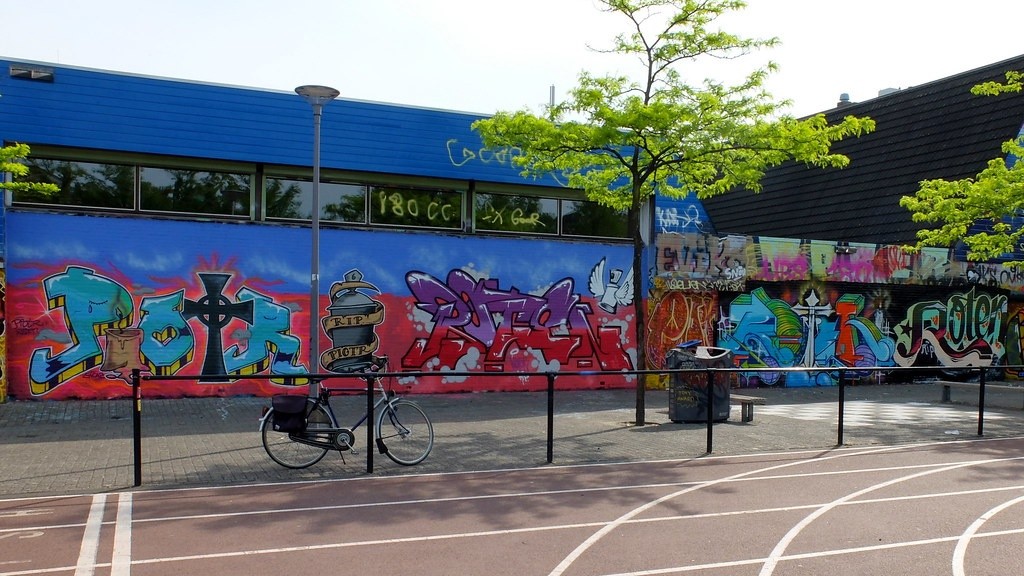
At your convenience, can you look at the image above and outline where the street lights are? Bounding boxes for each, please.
[296,85,341,439]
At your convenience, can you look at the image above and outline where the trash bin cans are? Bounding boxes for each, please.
[666,345,731,422]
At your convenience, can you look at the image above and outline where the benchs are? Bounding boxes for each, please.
[729,395,766,423]
[935,380,1024,410]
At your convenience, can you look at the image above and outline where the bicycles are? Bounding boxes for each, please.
[258,355,434,468]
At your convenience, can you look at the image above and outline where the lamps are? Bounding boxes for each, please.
[835,246,857,254]
[9,66,54,80]
